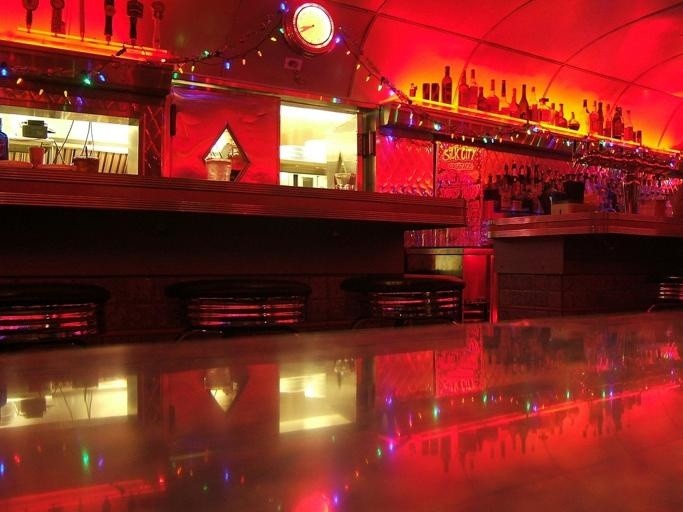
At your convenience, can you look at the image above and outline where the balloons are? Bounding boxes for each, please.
[72,97,87,113]
[77,70,108,99]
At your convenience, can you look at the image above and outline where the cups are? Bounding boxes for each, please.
[333,172,350,189]
[29,147,44,168]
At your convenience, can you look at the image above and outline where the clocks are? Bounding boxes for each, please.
[283,1,338,59]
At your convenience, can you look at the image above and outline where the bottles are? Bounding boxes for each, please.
[480,222,488,242]
[487,166,676,217]
[0,118,8,161]
[422,66,643,145]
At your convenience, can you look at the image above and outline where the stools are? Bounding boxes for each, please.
[646,275,683,315]
[167,280,314,334]
[2,280,112,339]
[342,275,466,328]
